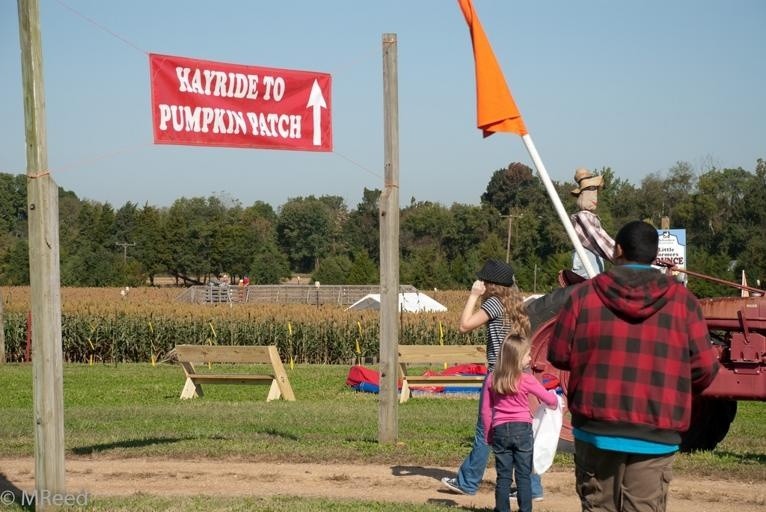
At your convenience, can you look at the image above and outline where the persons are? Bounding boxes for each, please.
[567,167,616,283]
[547,221,721,511]
[218,273,249,300]
[297,274,300,284]
[483,333,559,512]
[438,260,545,500]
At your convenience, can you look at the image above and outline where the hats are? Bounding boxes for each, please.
[570,170,607,196]
[474,259,514,287]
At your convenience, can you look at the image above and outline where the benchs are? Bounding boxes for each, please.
[396,343,490,406]
[173,343,297,403]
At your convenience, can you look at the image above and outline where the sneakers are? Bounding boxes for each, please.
[441,477,475,496]
[509,487,544,502]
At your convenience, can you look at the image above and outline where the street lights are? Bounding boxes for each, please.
[533,263,541,294]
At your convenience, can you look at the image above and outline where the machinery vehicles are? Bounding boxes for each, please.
[521,256,766,453]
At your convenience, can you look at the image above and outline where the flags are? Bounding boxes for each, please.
[459,0,525,140]
